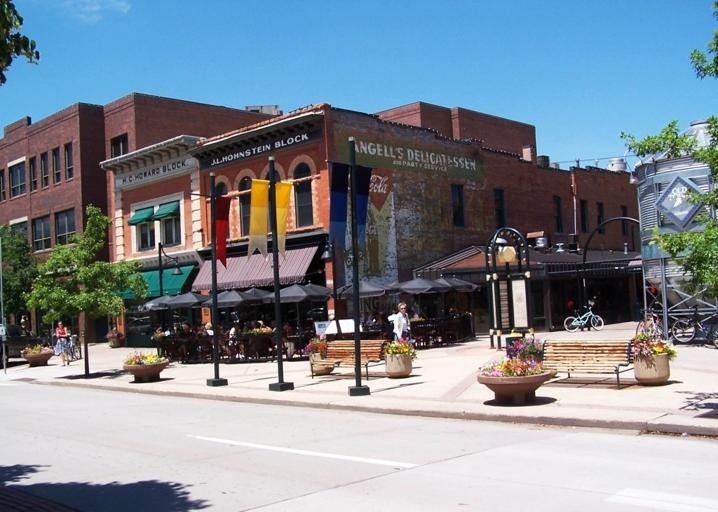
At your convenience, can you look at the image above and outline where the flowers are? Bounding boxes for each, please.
[384,340,416,359]
[20,343,53,355]
[304,339,329,352]
[123,351,170,365]
[626,334,676,359]
[476,332,548,377]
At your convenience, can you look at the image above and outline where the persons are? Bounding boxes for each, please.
[153,318,295,364]
[392,302,411,343]
[20,315,31,335]
[54,320,70,367]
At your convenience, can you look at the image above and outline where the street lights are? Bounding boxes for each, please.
[158,241,182,329]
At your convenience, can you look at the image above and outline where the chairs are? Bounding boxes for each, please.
[157,324,313,362]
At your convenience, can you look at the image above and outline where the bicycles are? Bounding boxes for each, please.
[636,307,664,340]
[562,303,604,333]
[63,336,83,362]
[670,303,718,350]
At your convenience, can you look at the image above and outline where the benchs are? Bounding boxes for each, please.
[323,340,387,380]
[540,340,634,390]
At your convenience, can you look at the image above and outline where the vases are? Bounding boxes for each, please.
[21,352,53,366]
[478,373,556,403]
[310,352,323,365]
[386,353,413,377]
[124,360,169,382]
[632,353,671,384]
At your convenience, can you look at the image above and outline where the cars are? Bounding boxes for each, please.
[0,323,49,369]
[126,311,188,337]
[306,308,324,321]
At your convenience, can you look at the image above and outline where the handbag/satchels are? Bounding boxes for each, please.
[402,329,410,341]
